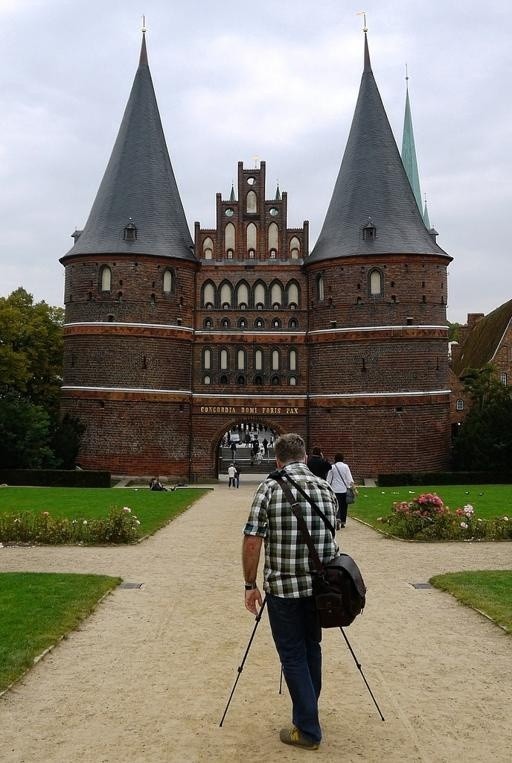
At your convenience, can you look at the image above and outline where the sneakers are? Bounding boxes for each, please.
[280,729,318,750]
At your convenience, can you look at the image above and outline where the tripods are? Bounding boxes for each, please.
[219,594,387,727]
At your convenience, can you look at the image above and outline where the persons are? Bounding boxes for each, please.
[225,433,274,466]
[227,463,237,489]
[241,431,342,751]
[234,464,241,488]
[325,452,358,530]
[149,477,176,491]
[307,447,332,480]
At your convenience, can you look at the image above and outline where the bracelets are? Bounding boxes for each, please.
[244,582,258,590]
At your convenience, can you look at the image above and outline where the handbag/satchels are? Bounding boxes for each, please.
[346,487,355,504]
[313,555,366,628]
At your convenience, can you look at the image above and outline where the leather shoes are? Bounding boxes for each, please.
[337,523,345,530]
